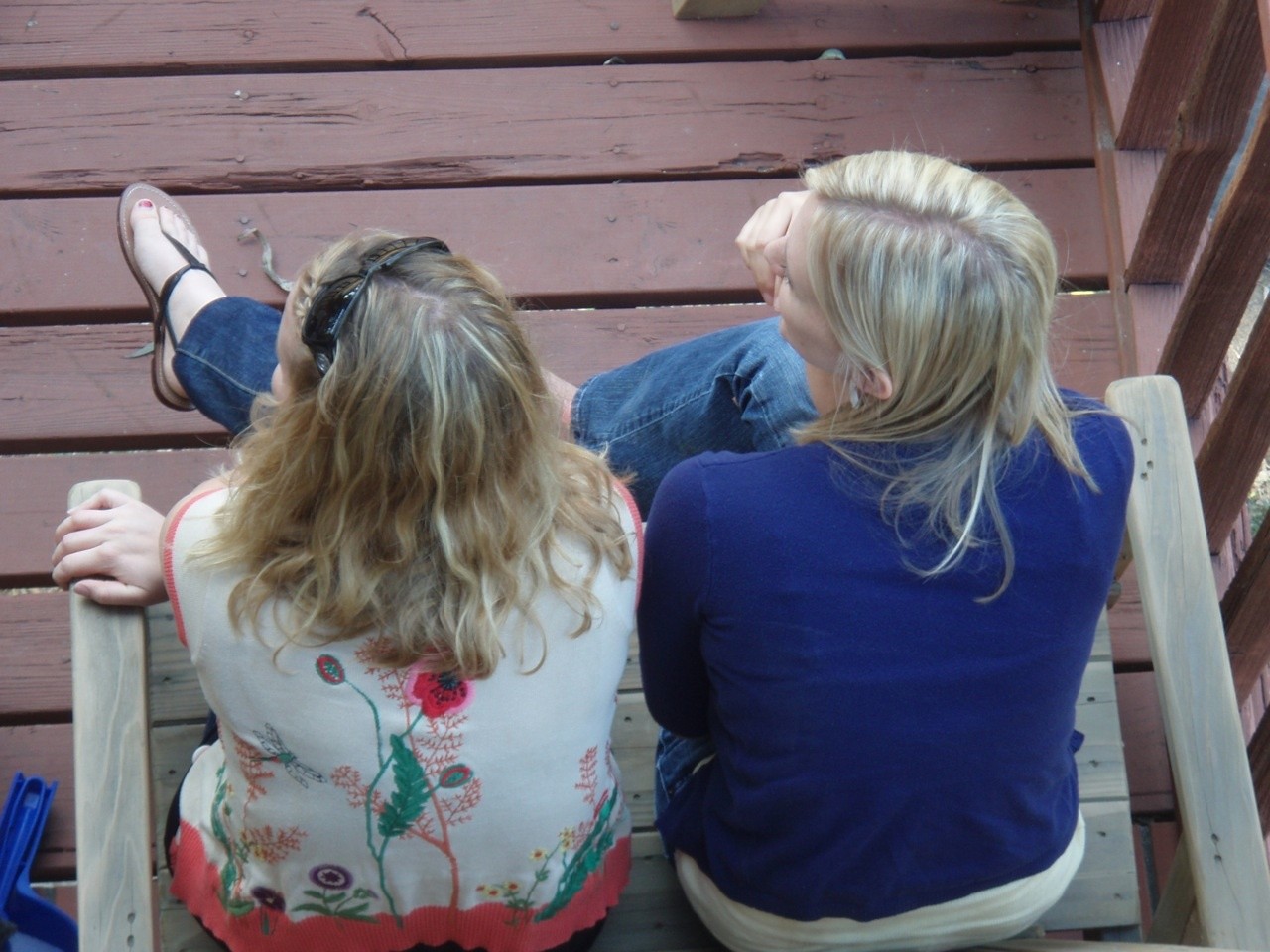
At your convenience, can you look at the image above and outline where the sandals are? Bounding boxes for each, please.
[117,183,222,411]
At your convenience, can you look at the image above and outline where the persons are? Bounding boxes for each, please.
[540,150,1137,952]
[49,184,646,952]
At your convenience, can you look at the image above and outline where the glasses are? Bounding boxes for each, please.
[301,235,454,377]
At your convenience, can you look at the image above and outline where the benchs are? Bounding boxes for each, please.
[68,375,1270,952]
